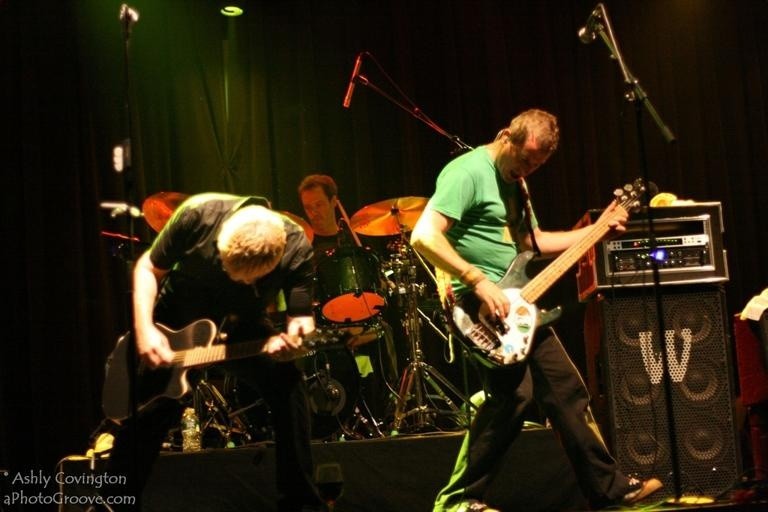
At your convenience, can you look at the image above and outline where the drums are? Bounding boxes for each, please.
[313,244,390,326]
[223,303,361,443]
[384,240,438,303]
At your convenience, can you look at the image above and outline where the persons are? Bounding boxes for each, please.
[407,108,667,512]
[298,174,396,324]
[88,190,331,511]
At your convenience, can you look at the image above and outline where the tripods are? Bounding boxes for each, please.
[384,262,480,432]
[191,374,251,448]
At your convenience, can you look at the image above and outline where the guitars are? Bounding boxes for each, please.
[102,317,350,427]
[445,177,648,369]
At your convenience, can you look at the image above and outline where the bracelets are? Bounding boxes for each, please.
[459,265,487,287]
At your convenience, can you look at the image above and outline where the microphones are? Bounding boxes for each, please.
[576,7,605,45]
[121,4,140,29]
[342,47,363,108]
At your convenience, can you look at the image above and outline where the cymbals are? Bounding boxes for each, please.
[140,192,197,235]
[352,195,430,237]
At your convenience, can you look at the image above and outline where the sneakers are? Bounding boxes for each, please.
[433,500,498,512]
[611,476,664,505]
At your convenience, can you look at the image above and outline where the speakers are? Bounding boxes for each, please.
[600,280,747,501]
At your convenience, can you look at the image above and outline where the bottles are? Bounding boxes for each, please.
[180,408,203,455]
